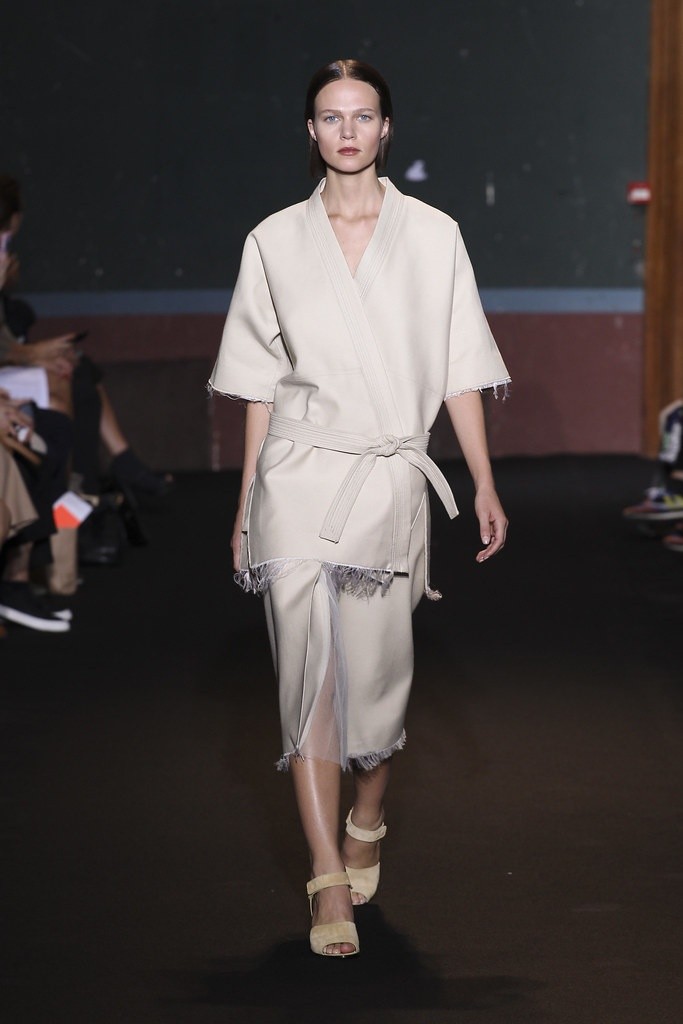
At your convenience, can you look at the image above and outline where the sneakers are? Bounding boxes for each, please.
[31,593,76,624]
[621,490,682,521]
[661,524,682,553]
[0,584,70,635]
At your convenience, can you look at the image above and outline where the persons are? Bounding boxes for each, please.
[0,168,173,640]
[206,60,511,960]
[620,396,683,556]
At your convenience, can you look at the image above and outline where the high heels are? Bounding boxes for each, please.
[305,869,363,962]
[343,800,389,907]
